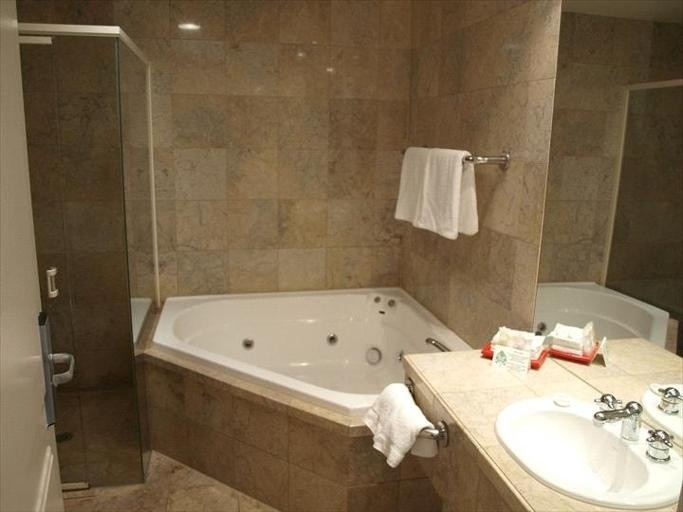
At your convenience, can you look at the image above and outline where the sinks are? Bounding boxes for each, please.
[642,383,683,439]
[494,396,683,510]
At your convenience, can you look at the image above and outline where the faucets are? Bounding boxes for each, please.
[657,386,683,416]
[594,401,643,441]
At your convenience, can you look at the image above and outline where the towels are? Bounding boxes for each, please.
[392,143,481,241]
[359,380,439,472]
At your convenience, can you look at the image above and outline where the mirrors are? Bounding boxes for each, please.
[530,0,683,453]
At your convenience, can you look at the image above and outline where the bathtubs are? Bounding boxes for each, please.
[144,286,476,431]
[129,296,159,355]
[531,280,679,356]
